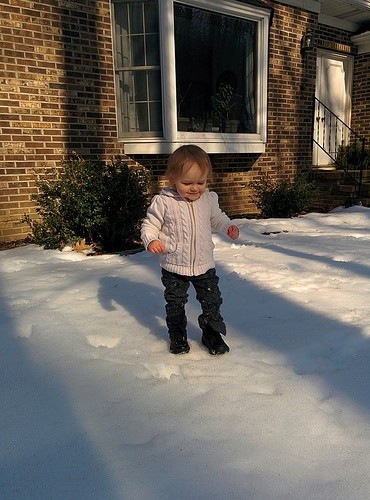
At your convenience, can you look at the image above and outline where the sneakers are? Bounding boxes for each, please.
[201,328,230,355]
[168,328,189,353]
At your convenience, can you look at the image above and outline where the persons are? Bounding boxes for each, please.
[140,144,240,356]
